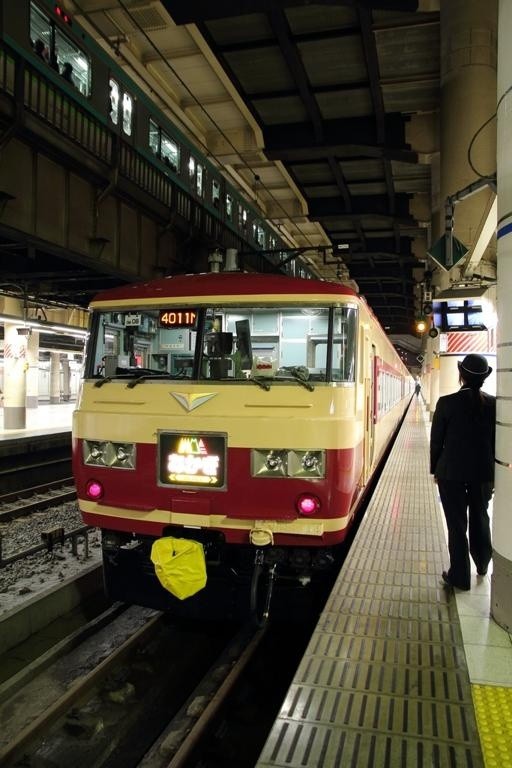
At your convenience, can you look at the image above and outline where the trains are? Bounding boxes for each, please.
[71,272,415,629]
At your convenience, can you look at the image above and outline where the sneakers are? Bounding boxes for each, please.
[477,545,493,576]
[442,569,471,591]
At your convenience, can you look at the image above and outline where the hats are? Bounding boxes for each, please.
[457,353,493,379]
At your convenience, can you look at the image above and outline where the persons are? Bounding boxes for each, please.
[415,383,421,396]
[31,37,180,177]
[430,352,496,589]
